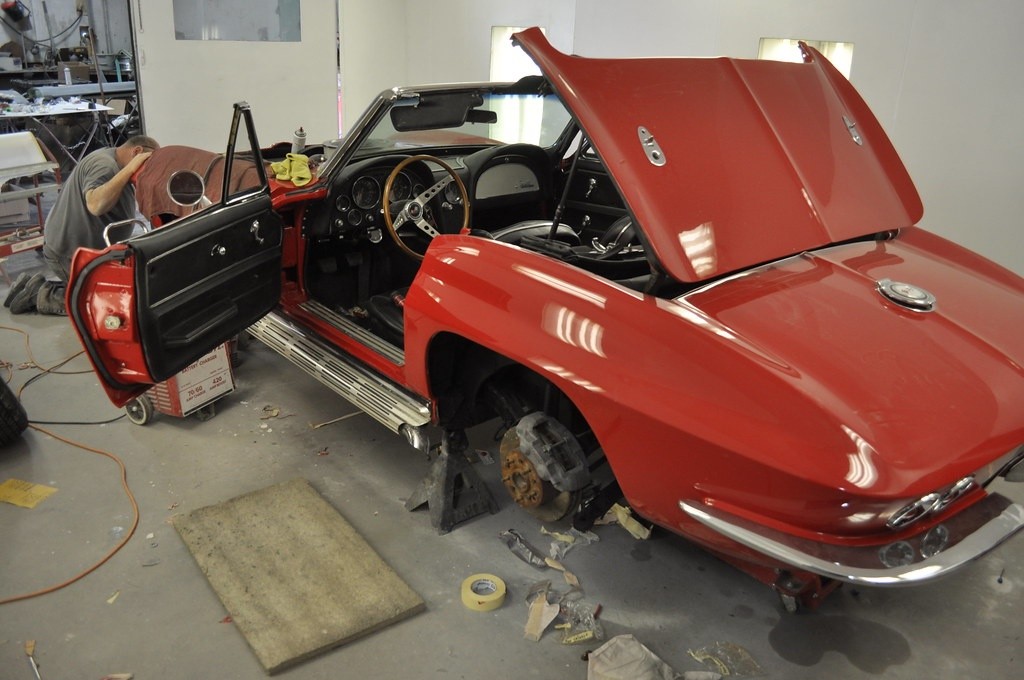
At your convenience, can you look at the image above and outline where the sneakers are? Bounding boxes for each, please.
[4,272,47,313]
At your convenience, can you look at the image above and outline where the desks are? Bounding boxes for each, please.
[0,98,114,170]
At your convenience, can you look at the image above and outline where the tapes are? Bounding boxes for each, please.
[461,573,506,611]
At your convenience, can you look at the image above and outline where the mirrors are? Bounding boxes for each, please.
[172,0,301,42]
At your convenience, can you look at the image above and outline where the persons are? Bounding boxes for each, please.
[3,135,160,316]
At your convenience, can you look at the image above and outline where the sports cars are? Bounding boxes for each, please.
[64,26,1023,618]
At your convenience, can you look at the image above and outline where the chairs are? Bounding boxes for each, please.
[598,211,639,249]
[487,219,581,246]
